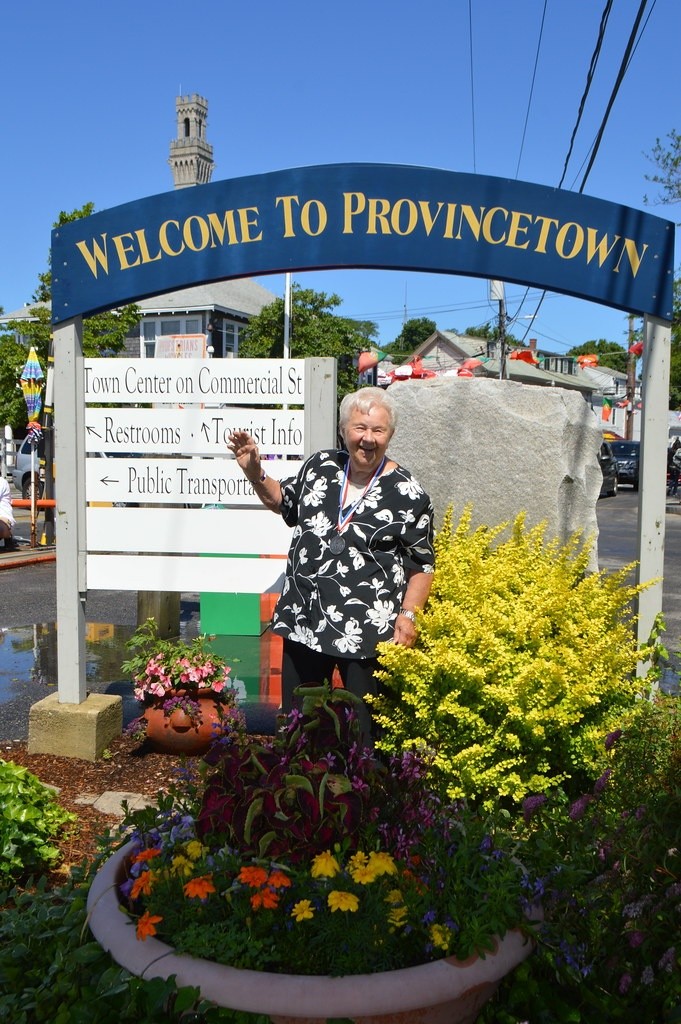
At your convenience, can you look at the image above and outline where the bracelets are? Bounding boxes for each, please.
[249,470,266,484]
[400,608,414,622]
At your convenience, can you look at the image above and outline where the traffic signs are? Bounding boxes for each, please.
[84,458,303,506]
[83,407,304,455]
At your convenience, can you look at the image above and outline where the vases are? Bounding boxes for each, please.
[86,814,545,1024]
[137,686,232,755]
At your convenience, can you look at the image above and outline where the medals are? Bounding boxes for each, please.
[330,536,345,555]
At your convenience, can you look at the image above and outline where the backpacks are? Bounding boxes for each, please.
[673,448,681,469]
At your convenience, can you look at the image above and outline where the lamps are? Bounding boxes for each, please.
[207,319,215,332]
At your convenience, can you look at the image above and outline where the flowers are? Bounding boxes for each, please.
[126,718,149,742]
[163,696,203,724]
[225,708,248,732]
[118,682,593,981]
[121,615,231,701]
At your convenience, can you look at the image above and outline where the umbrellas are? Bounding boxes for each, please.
[20,347,45,548]
[367,365,474,385]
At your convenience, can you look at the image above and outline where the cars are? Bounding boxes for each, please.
[597,441,620,497]
[12,427,55,500]
[668,425,681,449]
[610,440,640,491]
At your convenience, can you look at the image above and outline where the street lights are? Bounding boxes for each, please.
[500,315,538,380]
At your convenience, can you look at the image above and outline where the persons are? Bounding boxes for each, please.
[228,387,435,753]
[667,437,681,496]
[0,469,16,551]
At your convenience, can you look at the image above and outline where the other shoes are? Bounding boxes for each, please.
[667,488,671,496]
[4,534,13,551]
[673,493,677,497]
